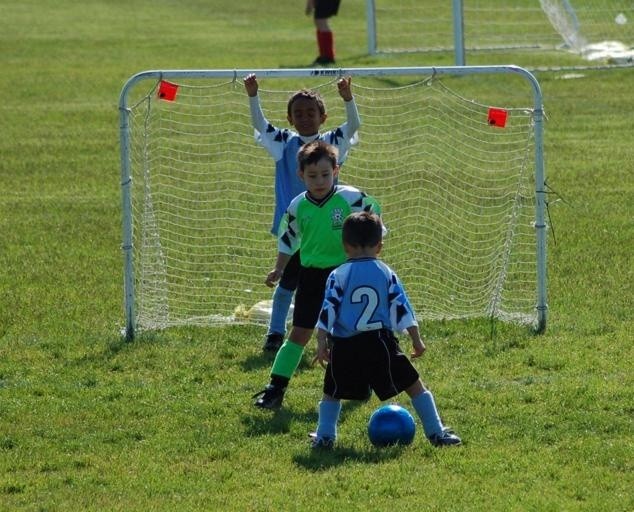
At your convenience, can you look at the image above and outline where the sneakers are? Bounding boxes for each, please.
[429,431,462,448]
[251,388,286,408]
[314,56,336,65]
[308,433,334,450]
[263,333,284,352]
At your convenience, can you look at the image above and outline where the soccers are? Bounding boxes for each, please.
[369,405,414,447]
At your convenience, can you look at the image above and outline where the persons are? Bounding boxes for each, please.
[243,71,362,351]
[254,144,389,407]
[310,212,462,451]
[301,0,344,67]
